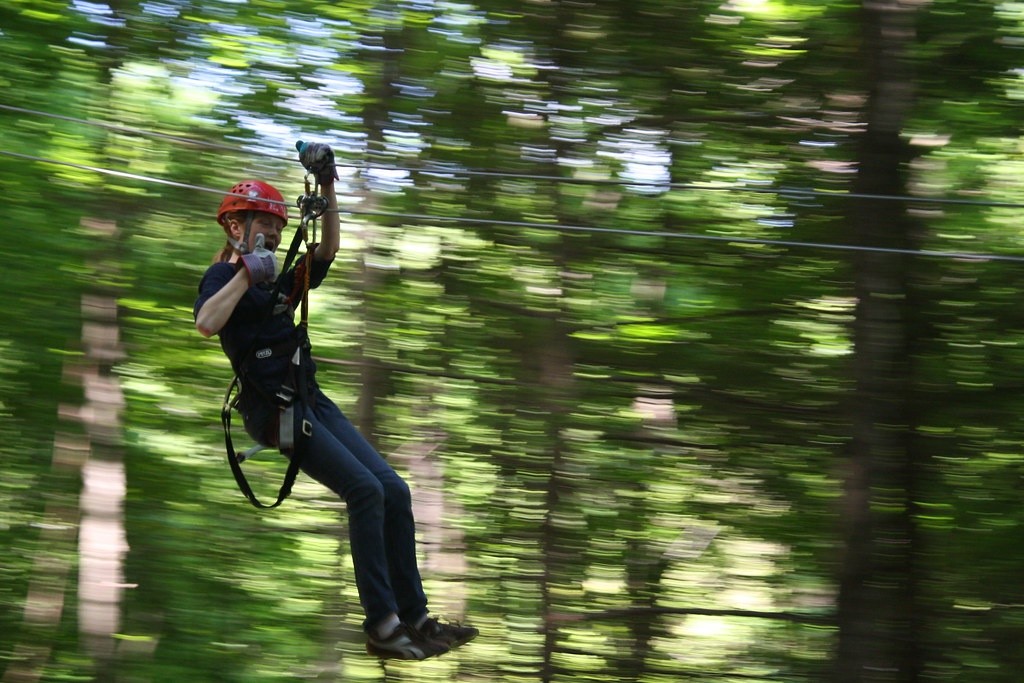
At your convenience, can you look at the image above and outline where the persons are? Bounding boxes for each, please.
[193,137,481,662]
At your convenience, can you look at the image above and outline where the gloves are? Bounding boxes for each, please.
[296,140,339,186]
[235,233,279,288]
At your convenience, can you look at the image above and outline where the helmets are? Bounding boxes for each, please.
[217,179,287,227]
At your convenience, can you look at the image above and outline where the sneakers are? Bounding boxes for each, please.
[419,618,479,648]
[366,622,449,660]
